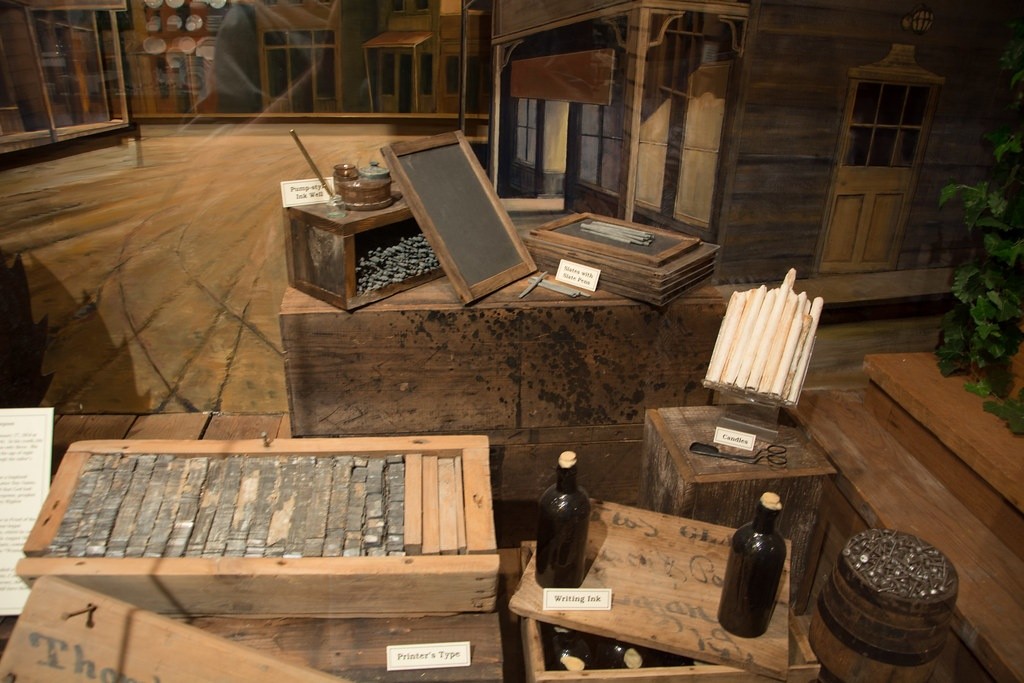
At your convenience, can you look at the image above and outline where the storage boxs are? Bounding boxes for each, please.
[510,494,821,683]
[17,436,499,617]
[282,179,445,311]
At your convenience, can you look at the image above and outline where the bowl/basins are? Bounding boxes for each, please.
[166,0,184,9]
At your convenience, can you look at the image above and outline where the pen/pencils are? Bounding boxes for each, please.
[289,128,343,211]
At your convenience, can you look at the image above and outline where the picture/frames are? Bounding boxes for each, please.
[381,129,538,307]
[522,210,721,306]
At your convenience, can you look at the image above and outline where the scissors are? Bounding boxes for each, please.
[689,442,788,466]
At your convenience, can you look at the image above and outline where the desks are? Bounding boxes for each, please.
[254,0,366,116]
[154,607,506,682]
[437,12,484,110]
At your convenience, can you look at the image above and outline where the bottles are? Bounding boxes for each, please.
[717,493,786,638]
[594,638,650,669]
[540,622,593,671]
[535,451,590,591]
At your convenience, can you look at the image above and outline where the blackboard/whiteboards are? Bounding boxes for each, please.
[379,129,538,305]
[520,211,722,308]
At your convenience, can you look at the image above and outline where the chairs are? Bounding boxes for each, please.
[360,1,441,113]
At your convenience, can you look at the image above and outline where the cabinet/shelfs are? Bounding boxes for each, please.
[636,405,838,617]
[277,262,729,508]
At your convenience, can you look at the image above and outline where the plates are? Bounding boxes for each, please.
[146,0,163,8]
[180,37,196,54]
[210,0,226,9]
[186,15,203,29]
[144,37,166,54]
[168,15,181,28]
